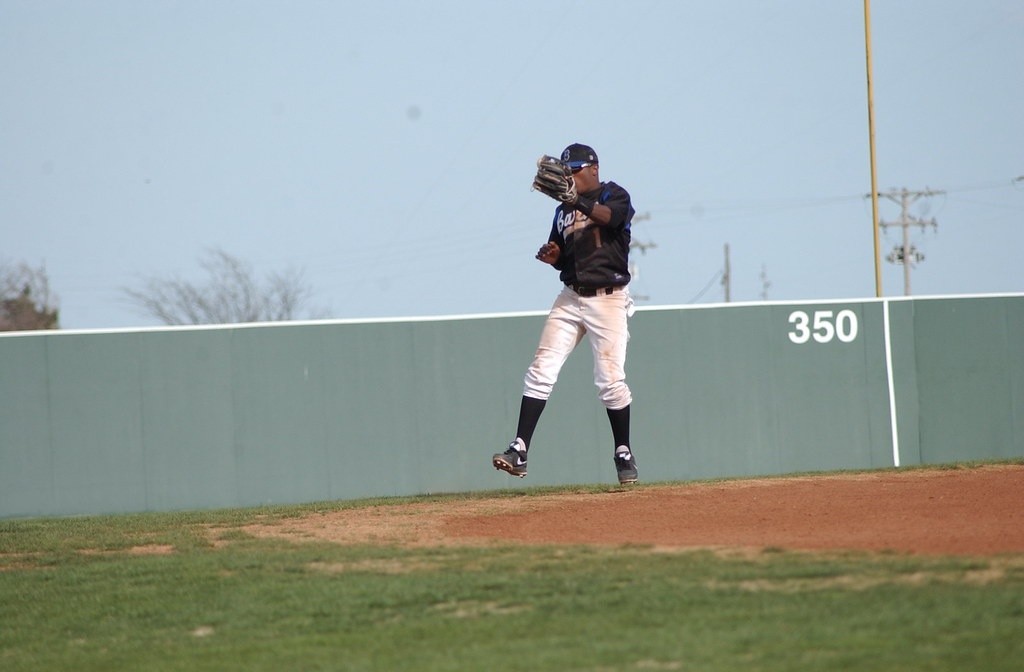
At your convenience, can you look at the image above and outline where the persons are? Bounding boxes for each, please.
[493,142,637,483]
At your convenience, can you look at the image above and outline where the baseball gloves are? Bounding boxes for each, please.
[529,154,578,204]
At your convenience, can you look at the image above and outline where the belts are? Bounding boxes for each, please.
[564,283,613,297]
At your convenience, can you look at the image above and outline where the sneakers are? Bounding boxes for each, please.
[613,451,638,483]
[492,447,528,478]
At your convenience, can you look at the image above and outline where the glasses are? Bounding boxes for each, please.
[571,163,591,175]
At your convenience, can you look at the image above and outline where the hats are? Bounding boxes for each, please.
[560,144,598,167]
